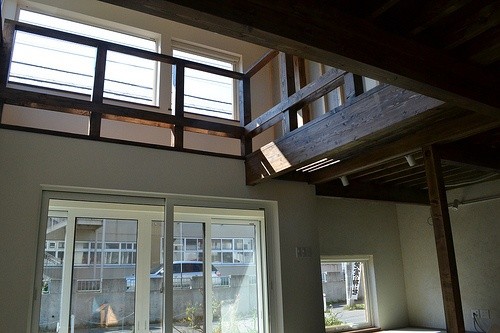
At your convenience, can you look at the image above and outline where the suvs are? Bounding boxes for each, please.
[126,260,221,288]
